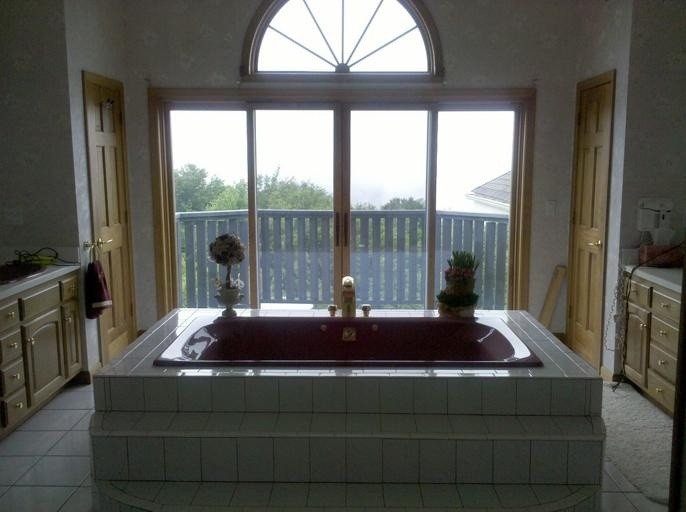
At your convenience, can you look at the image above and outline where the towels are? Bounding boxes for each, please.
[84,260,114,320]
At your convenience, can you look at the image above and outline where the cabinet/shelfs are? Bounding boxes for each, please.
[59,272,83,383]
[617,271,648,400]
[0,296,28,442]
[16,277,65,416]
[646,283,681,417]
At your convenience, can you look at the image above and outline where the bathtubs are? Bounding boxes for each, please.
[152,314,545,370]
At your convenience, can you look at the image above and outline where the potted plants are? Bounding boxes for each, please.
[435,292,477,321]
[441,247,480,295]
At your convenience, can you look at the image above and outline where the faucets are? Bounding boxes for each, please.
[340,276,356,318]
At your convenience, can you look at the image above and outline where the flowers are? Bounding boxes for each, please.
[207,230,246,290]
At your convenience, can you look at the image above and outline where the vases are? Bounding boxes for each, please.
[213,286,243,317]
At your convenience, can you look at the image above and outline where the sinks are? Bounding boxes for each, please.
[0,262,48,285]
